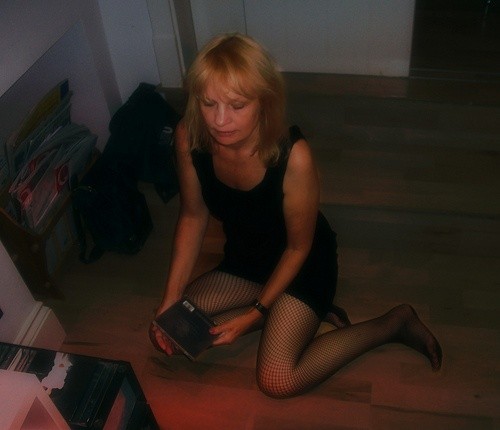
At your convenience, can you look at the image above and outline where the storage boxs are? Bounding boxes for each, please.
[0,367,71,430]
[0,149,101,282]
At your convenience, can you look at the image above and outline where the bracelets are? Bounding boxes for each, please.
[251,298,269,316]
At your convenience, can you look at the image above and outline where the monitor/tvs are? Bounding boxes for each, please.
[0,368,72,430]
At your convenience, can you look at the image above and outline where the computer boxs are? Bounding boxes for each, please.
[0,341,161,430]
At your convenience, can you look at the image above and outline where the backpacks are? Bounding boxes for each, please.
[70,158,153,262]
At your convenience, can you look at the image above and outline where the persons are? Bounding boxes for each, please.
[149,33,442,400]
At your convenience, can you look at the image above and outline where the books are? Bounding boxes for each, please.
[0,78,100,230]
